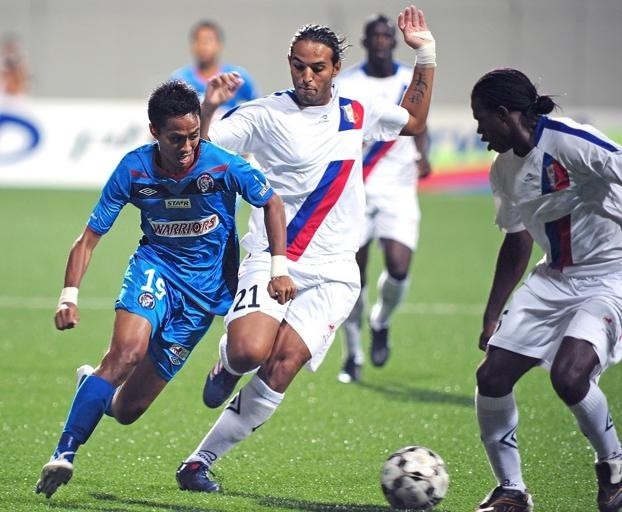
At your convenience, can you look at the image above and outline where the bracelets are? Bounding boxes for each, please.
[58,286,79,306]
[270,254,289,277]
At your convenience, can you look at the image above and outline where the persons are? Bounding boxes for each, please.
[173,1,438,494]
[35,81,299,498]
[331,10,431,383]
[469,67,621,511]
[167,18,259,125]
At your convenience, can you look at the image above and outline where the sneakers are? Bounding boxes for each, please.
[76,364,96,391]
[594,459,622,511]
[337,354,363,382]
[176,461,219,491]
[473,485,535,512]
[203,359,243,408]
[36,451,80,498]
[367,313,388,367]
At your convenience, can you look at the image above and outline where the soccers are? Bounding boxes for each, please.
[380,446,449,509]
